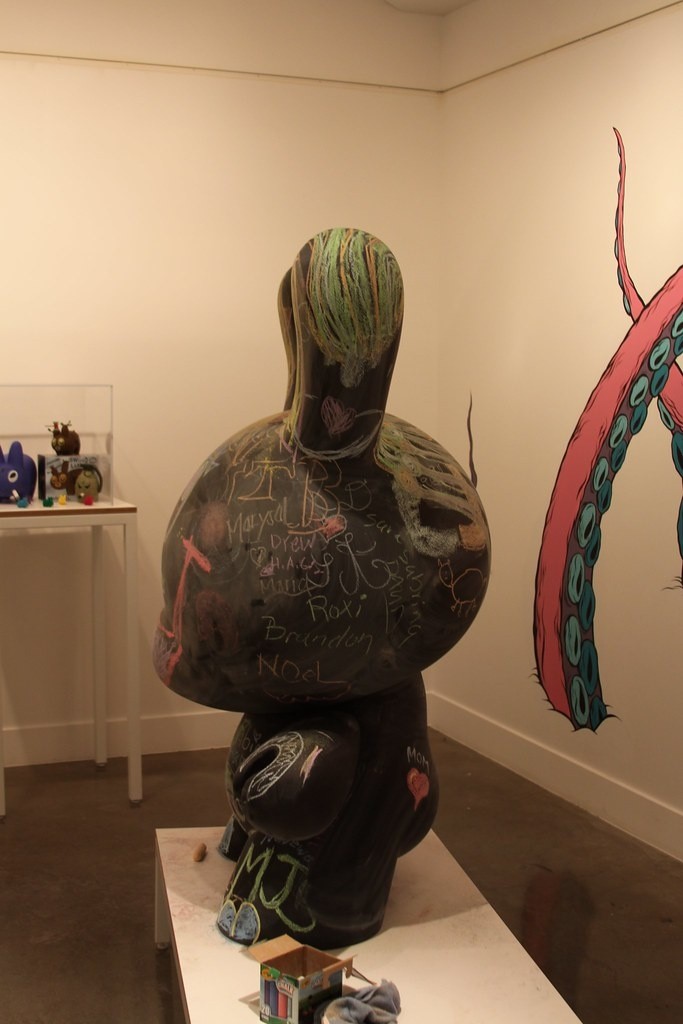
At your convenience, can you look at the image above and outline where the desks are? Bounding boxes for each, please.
[1,495,144,805]
[154,827,585,1024]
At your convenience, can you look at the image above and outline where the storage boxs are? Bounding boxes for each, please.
[247,933,375,1024]
